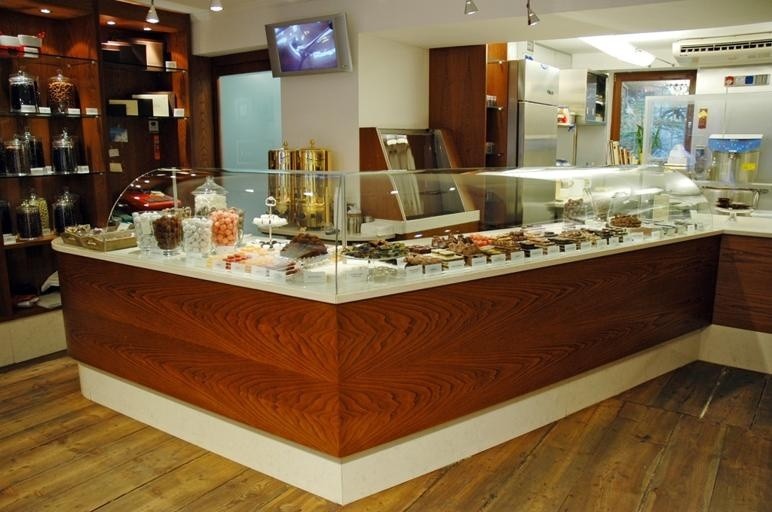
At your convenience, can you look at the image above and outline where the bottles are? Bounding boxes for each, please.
[0,125,79,176]
[190,175,231,215]
[8,65,43,115]
[0,183,82,243]
[46,68,78,116]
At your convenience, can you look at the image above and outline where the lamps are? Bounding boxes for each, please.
[464,1,480,14]
[210,1,223,12]
[526,0,540,26]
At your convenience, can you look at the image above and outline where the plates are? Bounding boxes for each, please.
[715,201,754,214]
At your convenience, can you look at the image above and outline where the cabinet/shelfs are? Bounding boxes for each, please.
[1,1,192,369]
[359,127,480,240]
[429,43,506,231]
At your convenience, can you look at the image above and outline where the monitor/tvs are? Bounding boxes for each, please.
[265,11,353,78]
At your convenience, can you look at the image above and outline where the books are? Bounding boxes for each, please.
[607,140,639,166]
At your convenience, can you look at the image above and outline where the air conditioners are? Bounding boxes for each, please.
[672,31,772,68]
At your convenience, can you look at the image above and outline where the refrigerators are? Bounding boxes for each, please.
[503,57,560,228]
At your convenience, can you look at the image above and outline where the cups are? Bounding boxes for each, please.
[132,207,246,268]
